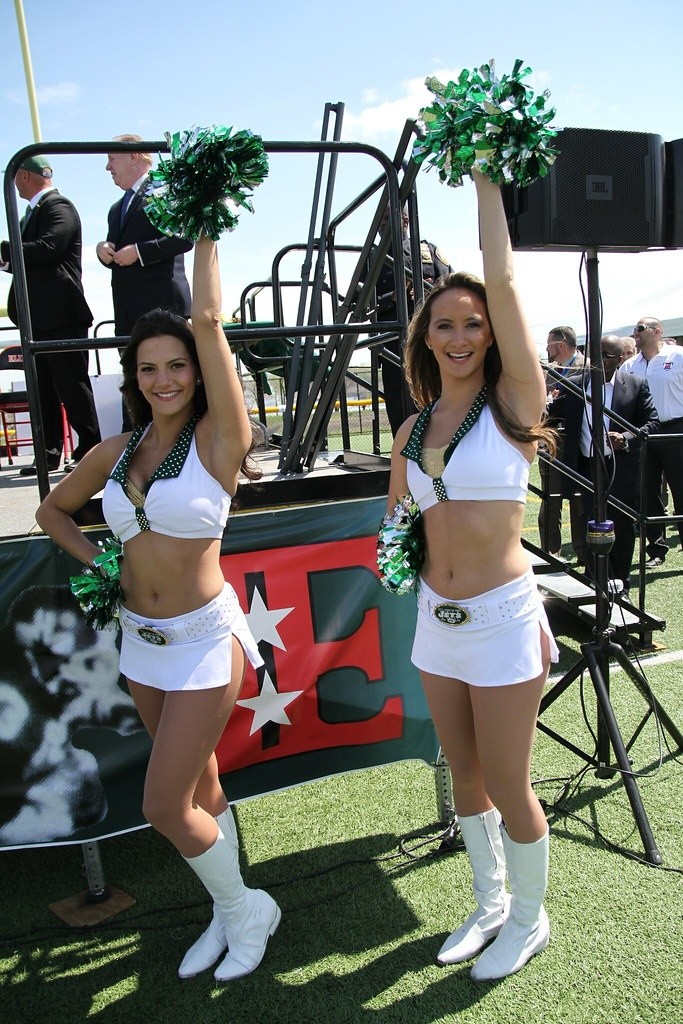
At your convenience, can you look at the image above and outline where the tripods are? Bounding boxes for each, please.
[534,250,683,866]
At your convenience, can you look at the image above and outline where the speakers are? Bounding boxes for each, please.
[477,127,683,253]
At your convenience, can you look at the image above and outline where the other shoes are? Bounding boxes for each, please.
[620,593,631,605]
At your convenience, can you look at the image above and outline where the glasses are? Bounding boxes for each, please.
[633,325,659,334]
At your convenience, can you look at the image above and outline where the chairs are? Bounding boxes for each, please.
[0,345,74,471]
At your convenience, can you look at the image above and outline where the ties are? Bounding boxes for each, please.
[117,188,135,228]
[21,204,33,237]
[554,364,563,381]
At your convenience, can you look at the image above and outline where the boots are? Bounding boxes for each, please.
[180,826,281,982]
[179,804,238,978]
[437,807,511,963]
[470,820,550,980]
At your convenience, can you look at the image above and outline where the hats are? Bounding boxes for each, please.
[3,156,54,179]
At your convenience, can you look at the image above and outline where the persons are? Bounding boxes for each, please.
[619,317,683,567]
[618,337,676,514]
[541,335,661,607]
[35,124,282,982]
[351,198,455,442]
[96,134,192,433]
[379,60,558,978]
[539,326,590,566]
[0,155,102,477]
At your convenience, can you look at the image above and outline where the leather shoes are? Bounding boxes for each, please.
[645,557,665,569]
[20,463,59,477]
[64,461,81,473]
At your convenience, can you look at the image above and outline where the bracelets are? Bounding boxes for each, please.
[623,436,627,447]
